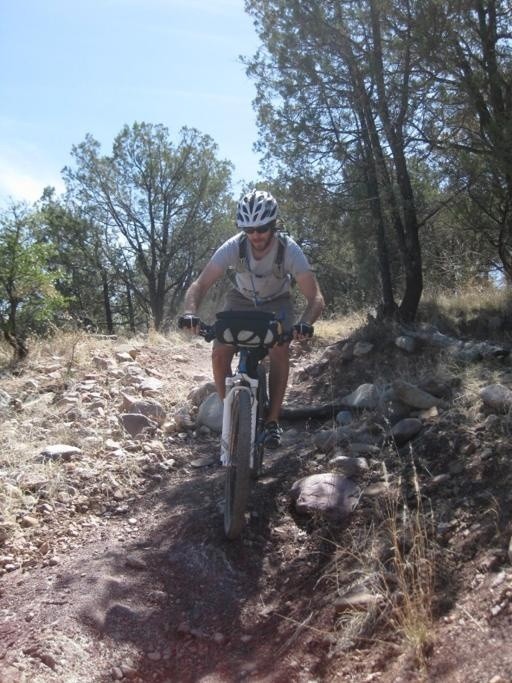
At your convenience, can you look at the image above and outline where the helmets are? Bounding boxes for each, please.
[236,189,279,227]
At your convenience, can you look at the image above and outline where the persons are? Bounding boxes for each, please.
[178,188,325,447]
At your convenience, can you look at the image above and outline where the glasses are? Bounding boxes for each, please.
[242,225,268,233]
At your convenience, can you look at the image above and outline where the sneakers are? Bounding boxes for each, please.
[264,420,282,449]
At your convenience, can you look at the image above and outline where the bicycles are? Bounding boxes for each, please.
[200,310,294,539]
